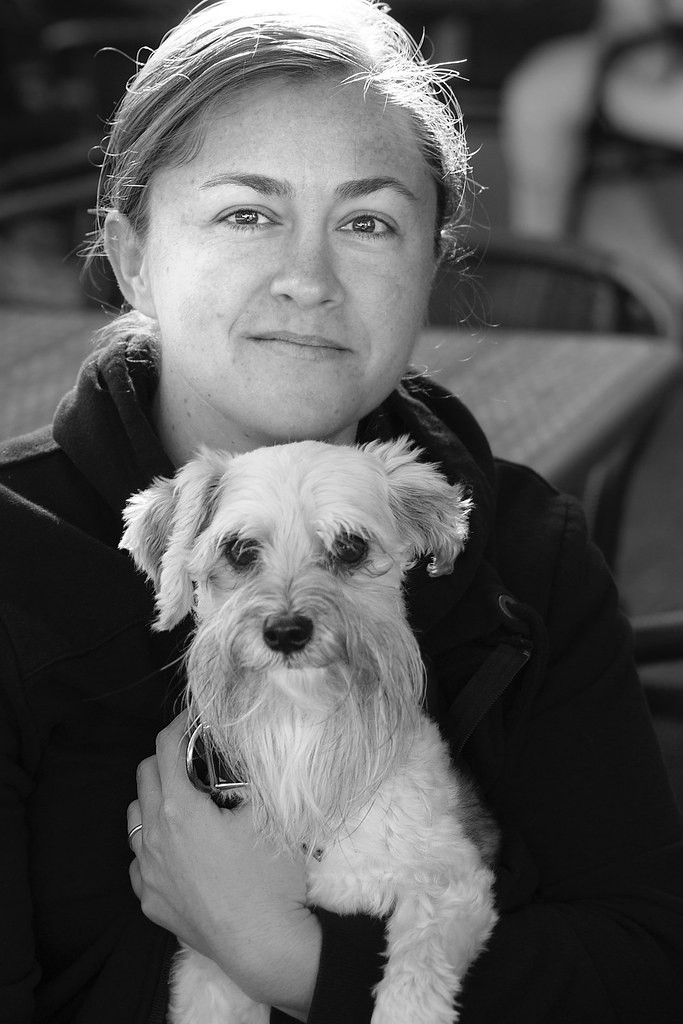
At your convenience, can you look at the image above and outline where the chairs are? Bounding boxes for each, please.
[569,13,683,262]
[408,234,677,543]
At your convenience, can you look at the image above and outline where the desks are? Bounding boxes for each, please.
[0,306,677,603]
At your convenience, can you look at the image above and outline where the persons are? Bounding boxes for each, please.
[0,0,682,1024]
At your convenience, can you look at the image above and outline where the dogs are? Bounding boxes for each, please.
[114,432,502,1023]
